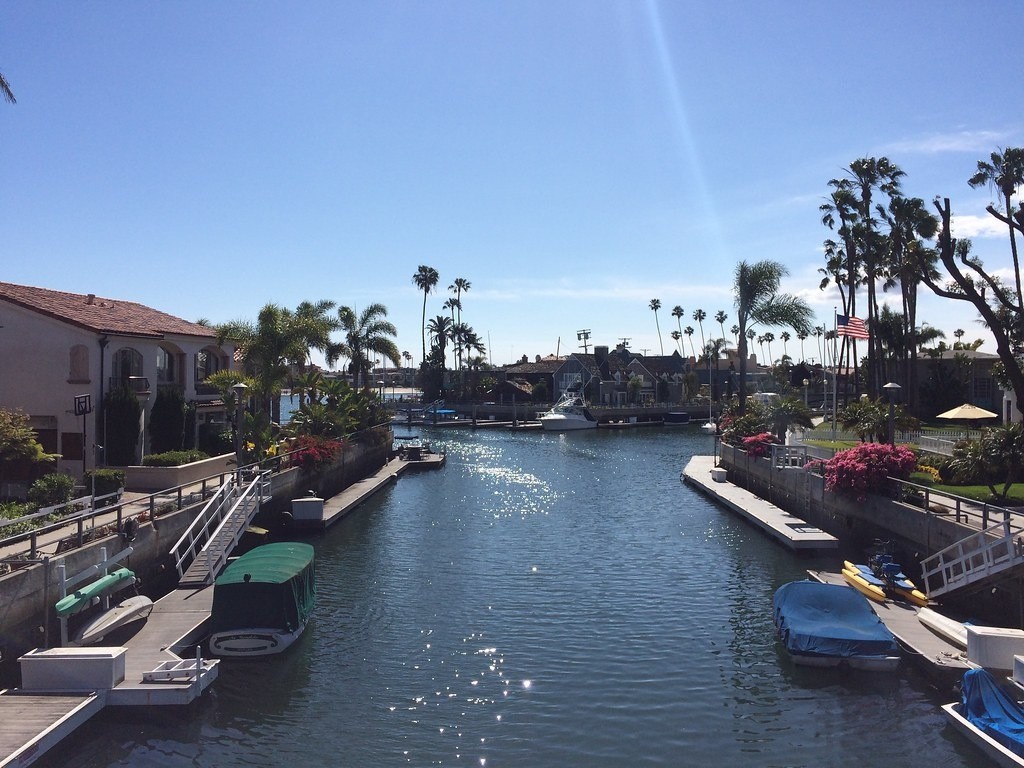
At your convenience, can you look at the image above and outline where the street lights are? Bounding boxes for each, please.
[883,381,902,447]
[233,383,248,486]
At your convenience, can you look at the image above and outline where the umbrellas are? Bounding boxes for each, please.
[937,404,998,438]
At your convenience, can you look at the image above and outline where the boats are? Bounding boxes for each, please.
[69,594,154,646]
[774,579,902,673]
[663,411,690,425]
[535,397,599,431]
[940,668,1024,768]
[841,538,929,610]
[210,542,317,657]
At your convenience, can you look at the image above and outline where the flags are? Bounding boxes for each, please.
[835,313,871,338]
